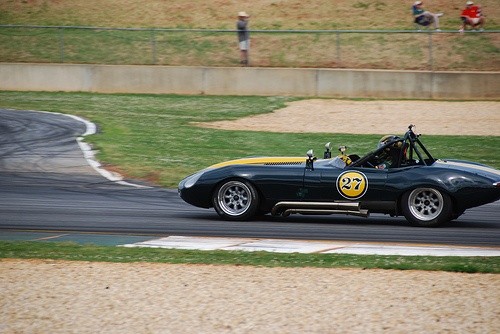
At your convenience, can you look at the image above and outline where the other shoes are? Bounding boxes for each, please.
[437,13,443,17]
[479,27,484,31]
[436,29,442,32]
[459,29,464,32]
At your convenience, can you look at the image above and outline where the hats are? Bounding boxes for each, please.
[237,12,250,18]
[466,1,474,5]
[415,1,423,5]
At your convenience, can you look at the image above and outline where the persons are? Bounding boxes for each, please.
[341,134,407,169]
[458,1,485,35]
[235,10,251,65]
[411,1,441,31]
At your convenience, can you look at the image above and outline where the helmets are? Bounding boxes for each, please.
[378,135,402,153]
